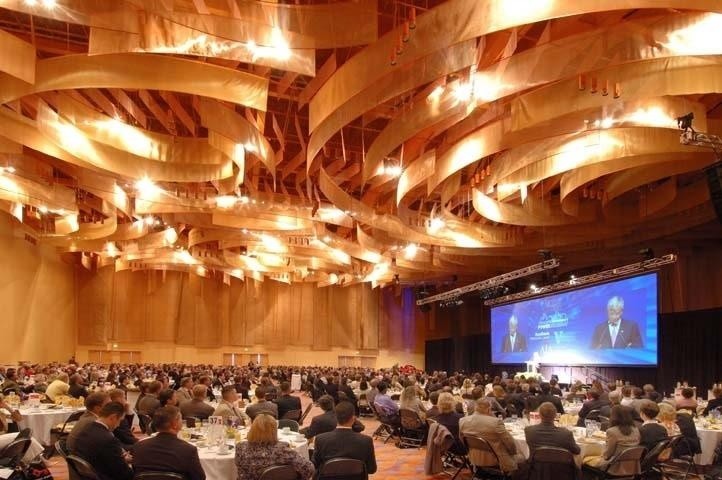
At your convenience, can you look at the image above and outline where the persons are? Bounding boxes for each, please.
[499,315,528,352]
[588,296,644,348]
[3,355,722,479]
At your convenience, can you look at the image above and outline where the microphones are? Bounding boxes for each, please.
[598,325,611,346]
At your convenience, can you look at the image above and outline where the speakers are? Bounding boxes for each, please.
[703,160,722,227]
[415,292,431,313]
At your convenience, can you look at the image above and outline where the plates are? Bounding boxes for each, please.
[296,440,306,442]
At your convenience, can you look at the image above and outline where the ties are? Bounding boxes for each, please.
[511,336,514,352]
[611,324,617,347]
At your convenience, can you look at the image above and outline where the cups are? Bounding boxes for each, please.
[511,415,517,424]
[568,397,583,408]
[56,394,86,409]
[283,426,291,434]
[297,434,305,441]
[554,420,601,439]
[33,408,40,412]
[697,397,702,405]
[708,410,720,423]
[498,415,502,420]
[181,417,253,454]
